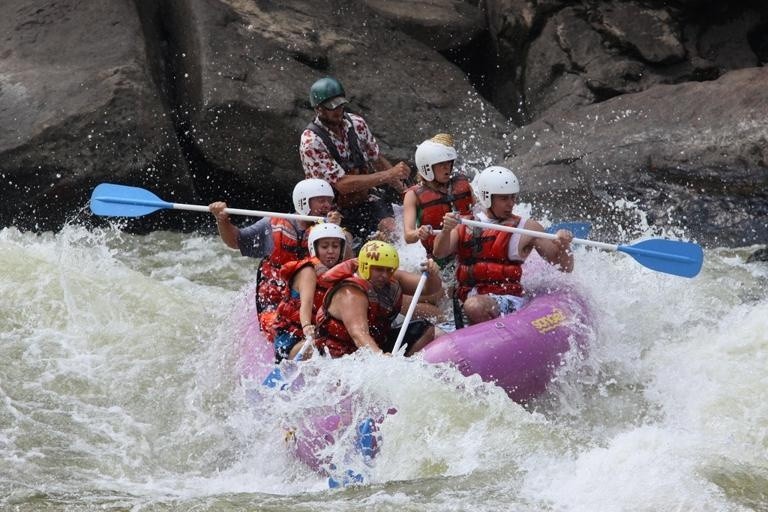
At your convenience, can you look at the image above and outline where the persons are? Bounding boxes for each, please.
[401,137,478,277]
[431,164,575,322]
[270,221,391,362]
[312,239,449,364]
[295,76,412,242]
[207,177,355,342]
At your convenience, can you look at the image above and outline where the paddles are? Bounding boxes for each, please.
[455,218,704,278]
[328,271,429,488]
[261,335,313,390]
[542,223,592,250]
[90,183,327,224]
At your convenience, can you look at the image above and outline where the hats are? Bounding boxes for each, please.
[322,97,348,110]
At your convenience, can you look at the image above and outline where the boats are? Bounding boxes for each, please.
[233,279,589,483]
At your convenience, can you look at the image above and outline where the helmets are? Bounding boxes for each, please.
[415,139,457,181]
[310,78,345,106]
[477,166,520,209]
[308,223,346,260]
[294,179,335,214]
[358,240,399,280]
